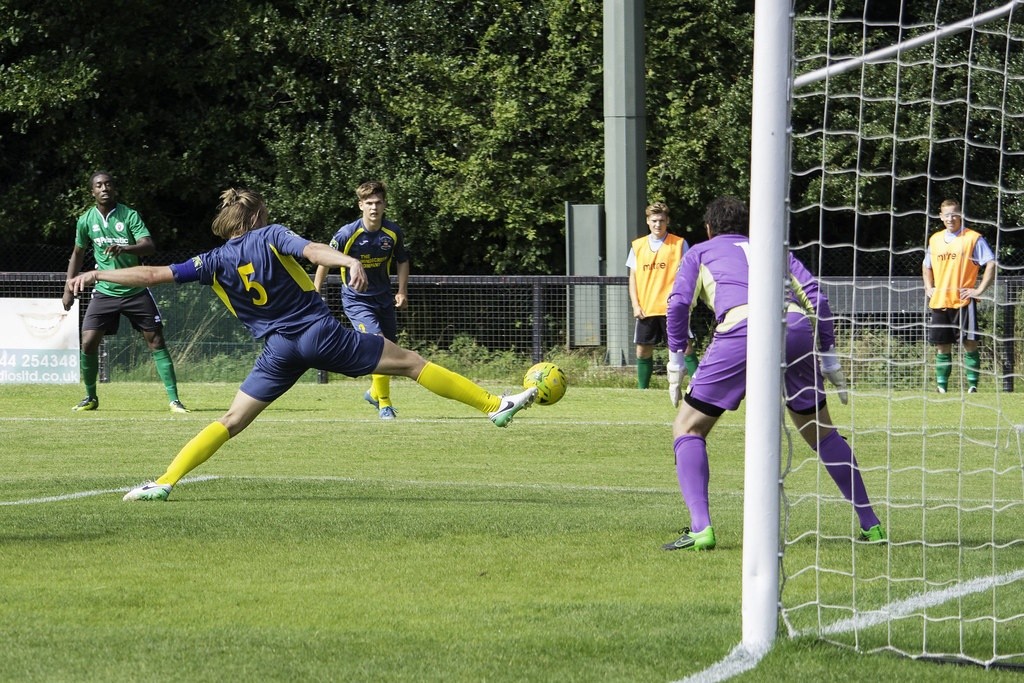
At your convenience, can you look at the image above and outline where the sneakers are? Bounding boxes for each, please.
[661,526,716,551]
[121,475,173,502]
[488,387,539,428]
[380,404,398,420]
[364,389,380,410]
[72,395,98,411]
[855,524,885,545]
[169,399,189,415]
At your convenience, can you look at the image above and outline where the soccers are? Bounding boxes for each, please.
[523,361,568,406]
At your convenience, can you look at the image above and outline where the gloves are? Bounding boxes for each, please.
[666,347,688,408]
[818,348,848,405]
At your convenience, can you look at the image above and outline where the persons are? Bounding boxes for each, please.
[68,186,539,502]
[314,183,409,420]
[624,201,700,388]
[62,170,192,413]
[922,200,996,392]
[658,197,888,551]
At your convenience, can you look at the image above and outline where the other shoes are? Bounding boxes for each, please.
[967,385,977,393]
[937,387,946,395]
